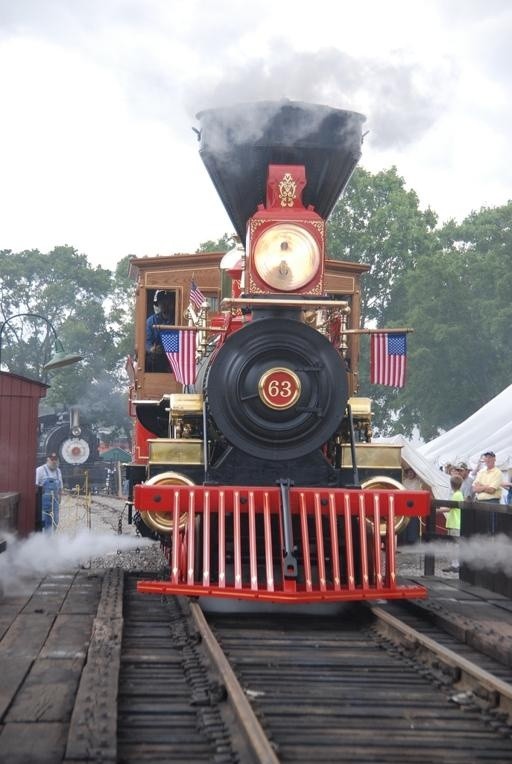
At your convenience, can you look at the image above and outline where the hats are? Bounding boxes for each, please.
[483,451,495,457]
[46,452,59,458]
[153,291,175,306]
[456,462,471,470]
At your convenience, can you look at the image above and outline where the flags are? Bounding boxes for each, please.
[189,282,205,310]
[370,333,406,388]
[160,329,196,386]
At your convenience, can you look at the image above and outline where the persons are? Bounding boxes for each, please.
[144,290,175,373]
[36,454,64,534]
[403,469,423,490]
[433,452,512,572]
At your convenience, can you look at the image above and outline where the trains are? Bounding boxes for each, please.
[125,100,432,616]
[36,407,106,494]
